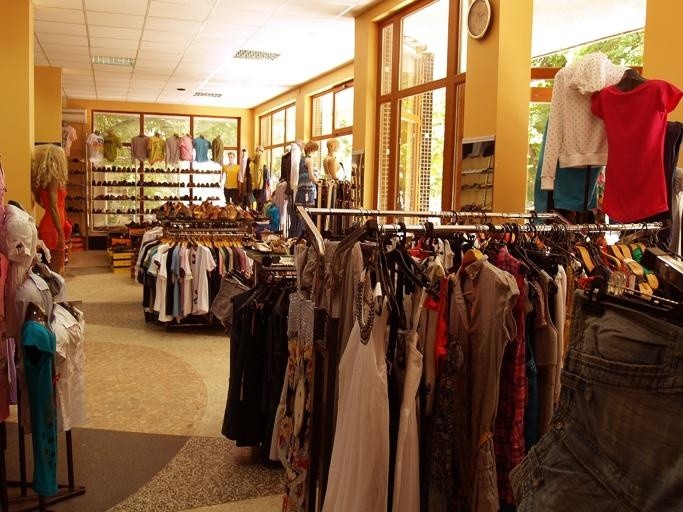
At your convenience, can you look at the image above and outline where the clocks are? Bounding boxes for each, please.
[464,0,492,42]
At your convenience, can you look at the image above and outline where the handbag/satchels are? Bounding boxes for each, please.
[218,206,238,221]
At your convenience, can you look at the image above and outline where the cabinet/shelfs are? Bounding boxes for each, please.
[64,156,87,215]
[90,162,226,232]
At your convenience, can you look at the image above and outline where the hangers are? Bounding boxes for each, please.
[158,230,246,252]
[288,207,658,317]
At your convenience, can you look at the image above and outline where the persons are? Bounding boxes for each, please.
[20,302,60,497]
[61,120,77,157]
[535,50,683,225]
[218,152,243,205]
[293,142,320,206]
[246,146,270,208]
[0,201,40,346]
[322,139,344,182]
[30,143,69,278]
[84,128,224,166]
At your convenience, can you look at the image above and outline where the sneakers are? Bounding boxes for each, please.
[90,165,223,215]
